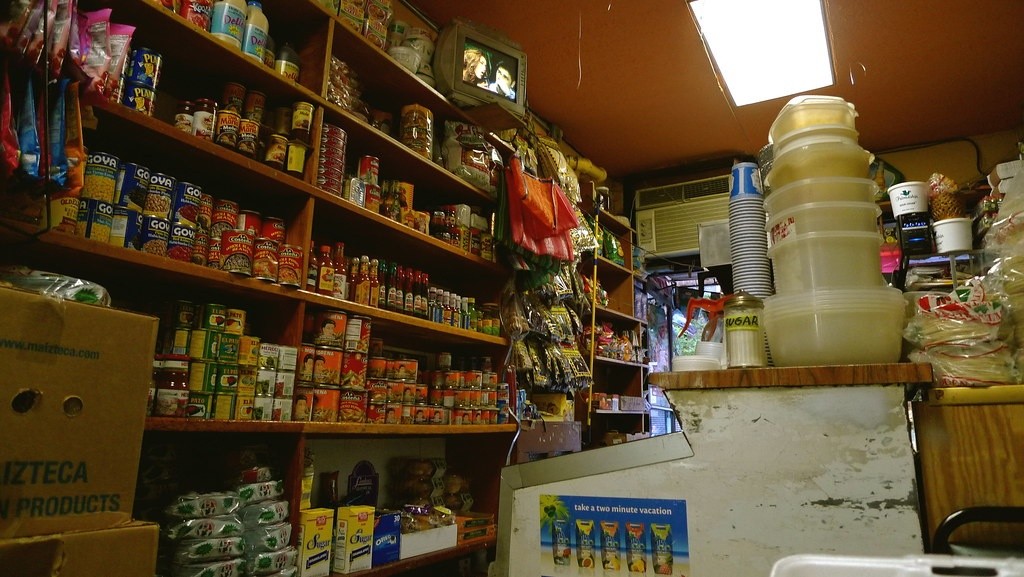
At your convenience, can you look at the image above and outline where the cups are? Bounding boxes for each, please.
[728,162,774,367]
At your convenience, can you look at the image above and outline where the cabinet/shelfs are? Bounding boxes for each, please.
[0,0,651,577]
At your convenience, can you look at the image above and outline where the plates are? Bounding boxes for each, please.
[672,355,721,370]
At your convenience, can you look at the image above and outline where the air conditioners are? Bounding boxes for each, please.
[634,175,733,260]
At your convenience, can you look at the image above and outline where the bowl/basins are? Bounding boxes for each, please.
[762,92,908,366]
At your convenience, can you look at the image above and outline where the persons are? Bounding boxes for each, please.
[478,59,517,100]
[464,47,487,86]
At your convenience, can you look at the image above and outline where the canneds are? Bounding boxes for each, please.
[38,46,315,289]
[145,299,298,423]
[292,307,511,425]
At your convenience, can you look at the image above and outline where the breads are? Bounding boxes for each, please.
[317,101,493,262]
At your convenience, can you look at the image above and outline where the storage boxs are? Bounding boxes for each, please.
[455,512,494,534]
[0,520,160,577]
[296,508,334,577]
[457,524,496,545]
[599,431,649,445]
[333,505,375,574]
[399,523,458,561]
[880,246,900,273]
[372,513,401,567]
[0,284,160,539]
[620,397,645,411]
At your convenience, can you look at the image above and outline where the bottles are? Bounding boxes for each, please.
[723,289,765,368]
[431,206,460,248]
[872,161,885,202]
[180,0,300,81]
[310,239,483,333]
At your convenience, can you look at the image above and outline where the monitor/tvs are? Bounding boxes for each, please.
[433,22,528,118]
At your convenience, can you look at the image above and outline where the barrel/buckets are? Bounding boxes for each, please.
[932,218,972,254]
[887,181,928,219]
